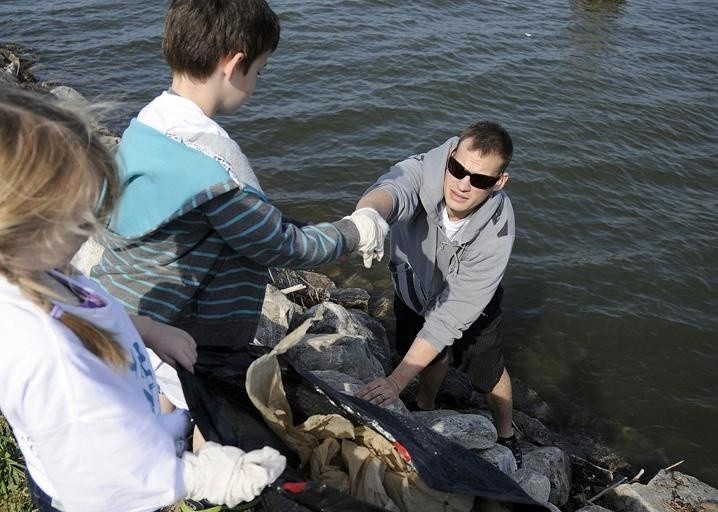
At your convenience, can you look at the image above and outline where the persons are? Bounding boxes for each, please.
[0,86,199,512]
[353,121,522,469]
[93,0,376,512]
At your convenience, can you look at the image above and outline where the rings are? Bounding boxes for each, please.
[377,394,385,403]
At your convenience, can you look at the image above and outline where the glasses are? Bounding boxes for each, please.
[447,147,506,191]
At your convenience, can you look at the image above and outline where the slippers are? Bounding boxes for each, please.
[494,432,523,471]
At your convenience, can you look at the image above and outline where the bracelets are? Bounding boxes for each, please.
[387,376,401,393]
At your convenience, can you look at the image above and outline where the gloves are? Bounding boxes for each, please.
[341,205,391,271]
[180,440,289,508]
